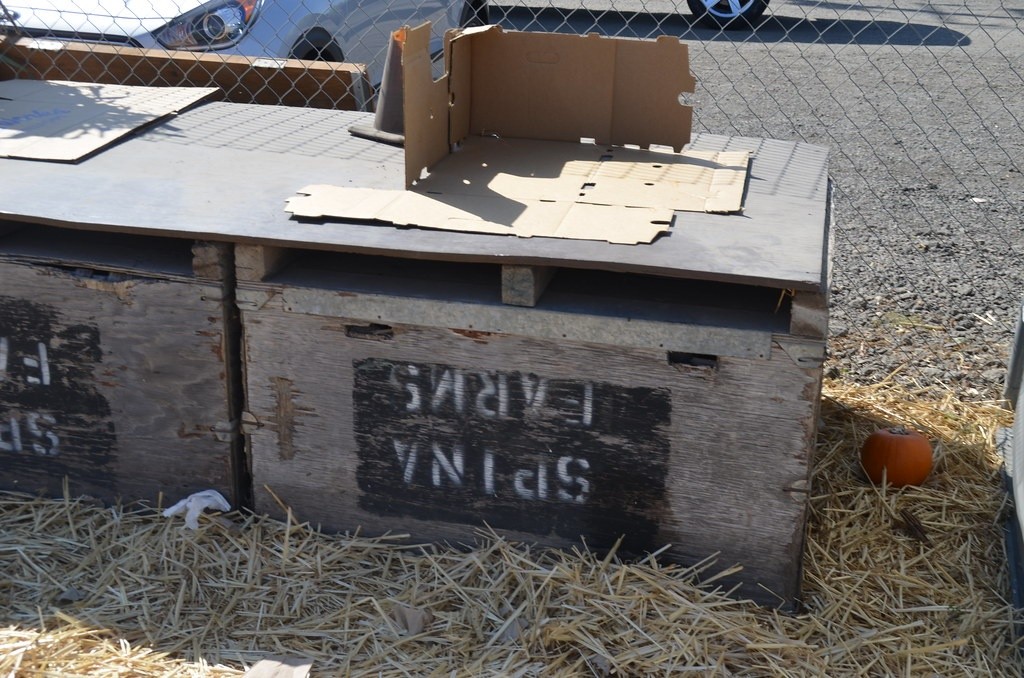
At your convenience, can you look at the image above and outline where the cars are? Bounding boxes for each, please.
[1,0,490,114]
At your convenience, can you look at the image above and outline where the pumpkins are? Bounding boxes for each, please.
[860,425,931,487]
